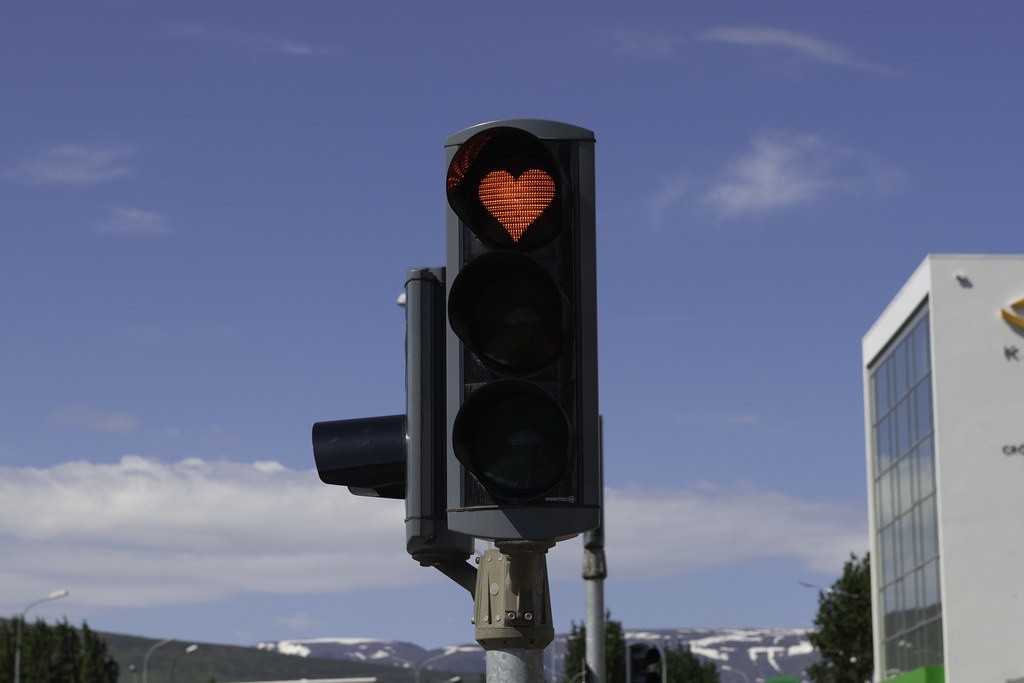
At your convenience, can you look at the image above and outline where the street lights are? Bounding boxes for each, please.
[170,643,198,683]
[14,590,70,683]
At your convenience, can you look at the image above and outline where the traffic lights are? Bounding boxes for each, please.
[310,412,409,502]
[438,114,599,553]
